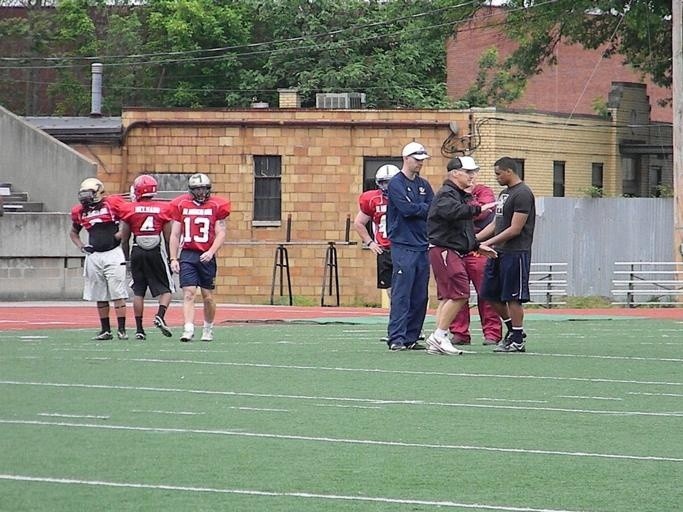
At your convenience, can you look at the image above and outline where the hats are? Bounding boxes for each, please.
[402,142,432,161]
[447,156,479,171]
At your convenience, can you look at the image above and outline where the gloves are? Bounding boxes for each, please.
[81,244,95,255]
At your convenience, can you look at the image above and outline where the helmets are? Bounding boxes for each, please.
[188,173,211,204]
[130,175,158,203]
[78,177,105,207]
[374,164,400,196]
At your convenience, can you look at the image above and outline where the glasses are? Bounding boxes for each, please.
[407,150,426,156]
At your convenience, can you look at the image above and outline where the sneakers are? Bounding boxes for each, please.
[200,327,214,341]
[135,332,146,340]
[90,330,113,340]
[152,314,172,337]
[379,329,525,356]
[116,329,129,340]
[180,329,194,342]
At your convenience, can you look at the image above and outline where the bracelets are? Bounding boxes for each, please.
[366,240,372,247]
[169,257,179,261]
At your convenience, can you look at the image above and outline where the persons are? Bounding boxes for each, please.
[156,172,232,342]
[351,163,426,341]
[448,172,503,347]
[425,154,501,359]
[118,173,173,340]
[384,140,433,351]
[474,155,536,352]
[68,176,129,340]
